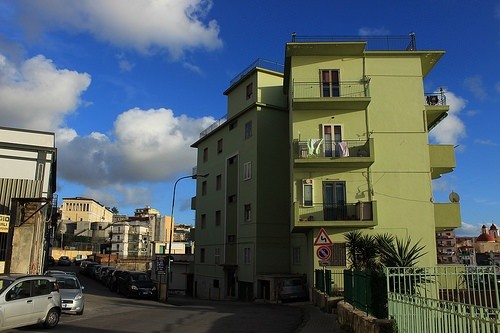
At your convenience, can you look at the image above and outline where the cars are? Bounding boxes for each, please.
[43,271,85,315]
[0,273,62,333]
[74,256,158,300]
[57,256,71,266]
[48,256,56,267]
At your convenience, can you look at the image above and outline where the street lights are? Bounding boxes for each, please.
[166,172,210,301]
